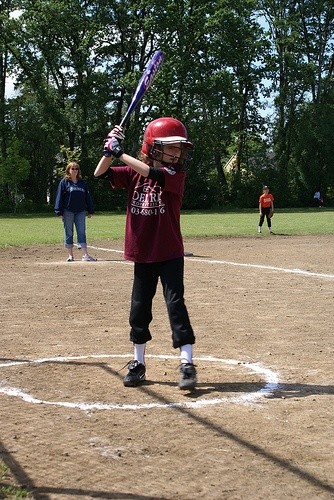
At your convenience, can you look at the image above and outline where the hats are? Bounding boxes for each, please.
[263,185,270,189]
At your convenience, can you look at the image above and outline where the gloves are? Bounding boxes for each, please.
[102,137,123,158]
[107,125,126,144]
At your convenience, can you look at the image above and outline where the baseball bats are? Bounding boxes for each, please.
[103,51,166,158]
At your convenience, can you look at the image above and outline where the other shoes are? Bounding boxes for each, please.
[82,254,97,261]
[257,228,261,233]
[67,255,73,261]
[268,229,273,234]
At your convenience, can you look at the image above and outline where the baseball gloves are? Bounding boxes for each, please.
[268,211,273,218]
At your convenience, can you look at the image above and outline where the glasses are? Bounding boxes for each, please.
[72,167,78,170]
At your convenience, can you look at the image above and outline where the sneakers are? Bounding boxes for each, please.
[124,359,146,387]
[178,363,197,389]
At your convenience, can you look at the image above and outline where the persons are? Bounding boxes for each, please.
[320,196,323,208]
[54,161,97,261]
[314,190,321,208]
[94,116,197,390]
[258,185,275,233]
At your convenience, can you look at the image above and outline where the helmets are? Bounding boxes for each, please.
[141,117,193,159]
